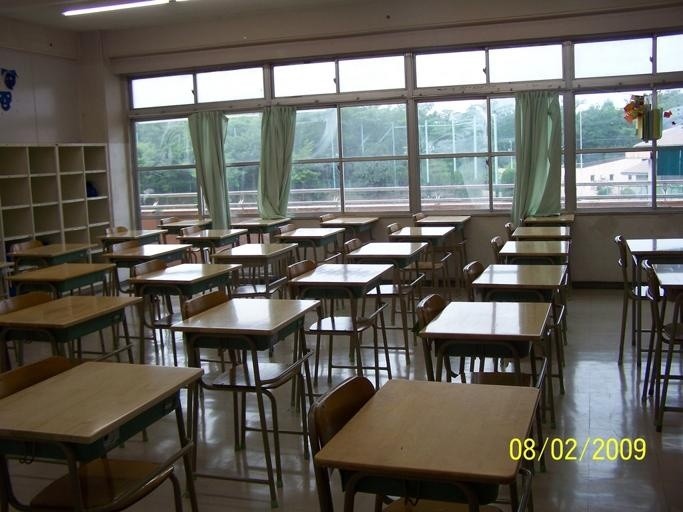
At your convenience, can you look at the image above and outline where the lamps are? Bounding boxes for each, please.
[60,0,191,17]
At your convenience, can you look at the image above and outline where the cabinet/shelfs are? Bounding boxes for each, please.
[0,141,115,315]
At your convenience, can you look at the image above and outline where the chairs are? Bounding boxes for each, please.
[614,233,680,435]
[0,211,576,510]
